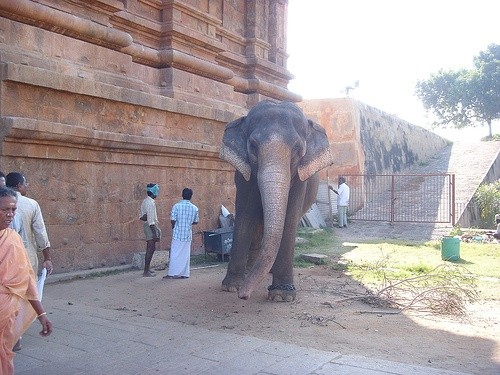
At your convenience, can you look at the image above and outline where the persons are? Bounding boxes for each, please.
[328,178,350,228]
[163,188,199,279]
[0,186,53,375]
[6,173,52,352]
[138,184,161,277]
[486,214,500,240]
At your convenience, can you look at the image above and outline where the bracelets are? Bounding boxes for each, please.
[38,311,46,320]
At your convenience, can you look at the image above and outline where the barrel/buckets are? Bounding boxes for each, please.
[441,237,460,261]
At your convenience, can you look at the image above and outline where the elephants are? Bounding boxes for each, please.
[219,99,334,302]
